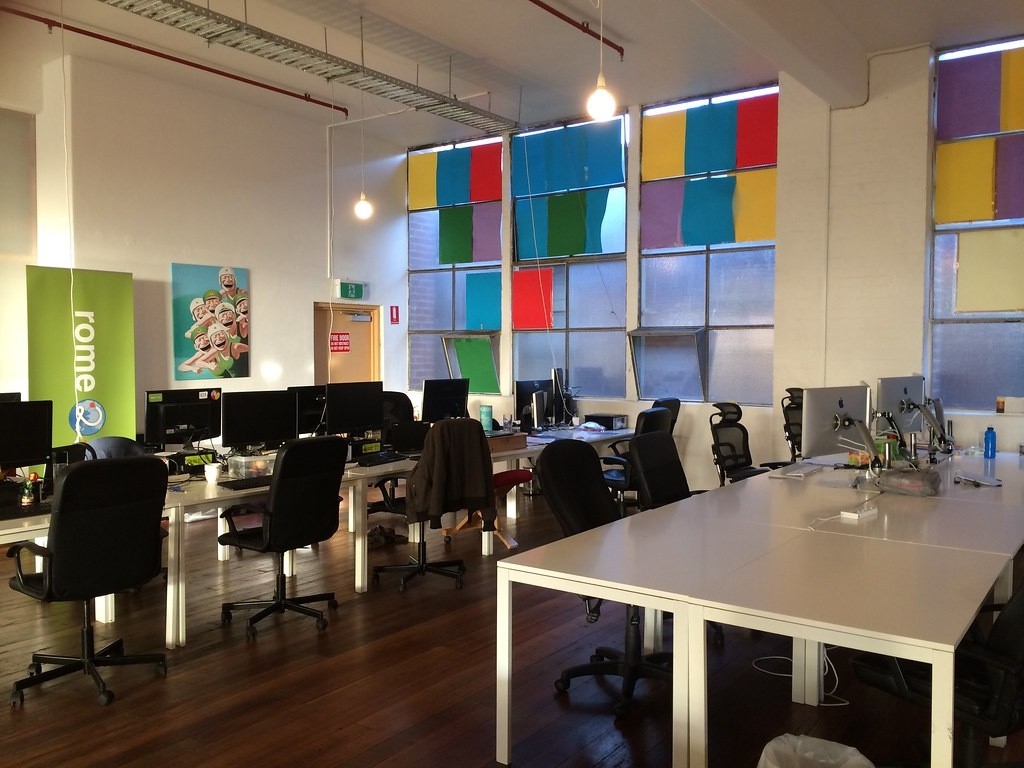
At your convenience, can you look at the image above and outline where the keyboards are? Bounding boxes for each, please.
[786,466,824,477]
[218,475,273,491]
[353,451,407,467]
[0,502,52,521]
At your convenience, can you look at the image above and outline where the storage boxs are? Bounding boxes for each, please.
[227,455,276,481]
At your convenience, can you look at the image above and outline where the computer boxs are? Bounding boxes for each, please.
[585,413,629,430]
[145,450,216,475]
[0,479,47,504]
[346,438,382,463]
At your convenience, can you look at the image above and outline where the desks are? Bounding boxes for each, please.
[495,451,1022,768]
[0,429,633,650]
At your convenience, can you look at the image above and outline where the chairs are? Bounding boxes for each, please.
[217,435,349,638]
[7,455,169,706]
[366,419,497,595]
[533,388,803,728]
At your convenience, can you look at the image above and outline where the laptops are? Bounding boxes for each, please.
[391,421,431,458]
[42,444,86,492]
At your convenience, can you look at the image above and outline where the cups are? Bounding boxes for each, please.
[572,417,580,427]
[480,405,492,430]
[503,413,512,430]
[520,414,532,435]
[204,462,222,483]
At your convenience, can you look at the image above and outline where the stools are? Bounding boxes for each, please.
[442,470,533,549]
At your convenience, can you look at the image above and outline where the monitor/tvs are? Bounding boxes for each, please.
[801,372,947,475]
[0,392,53,471]
[144,379,469,450]
[513,367,574,435]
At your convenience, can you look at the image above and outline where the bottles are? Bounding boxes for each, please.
[997,396,1005,413]
[984,427,996,459]
[887,434,898,459]
[22,489,35,507]
